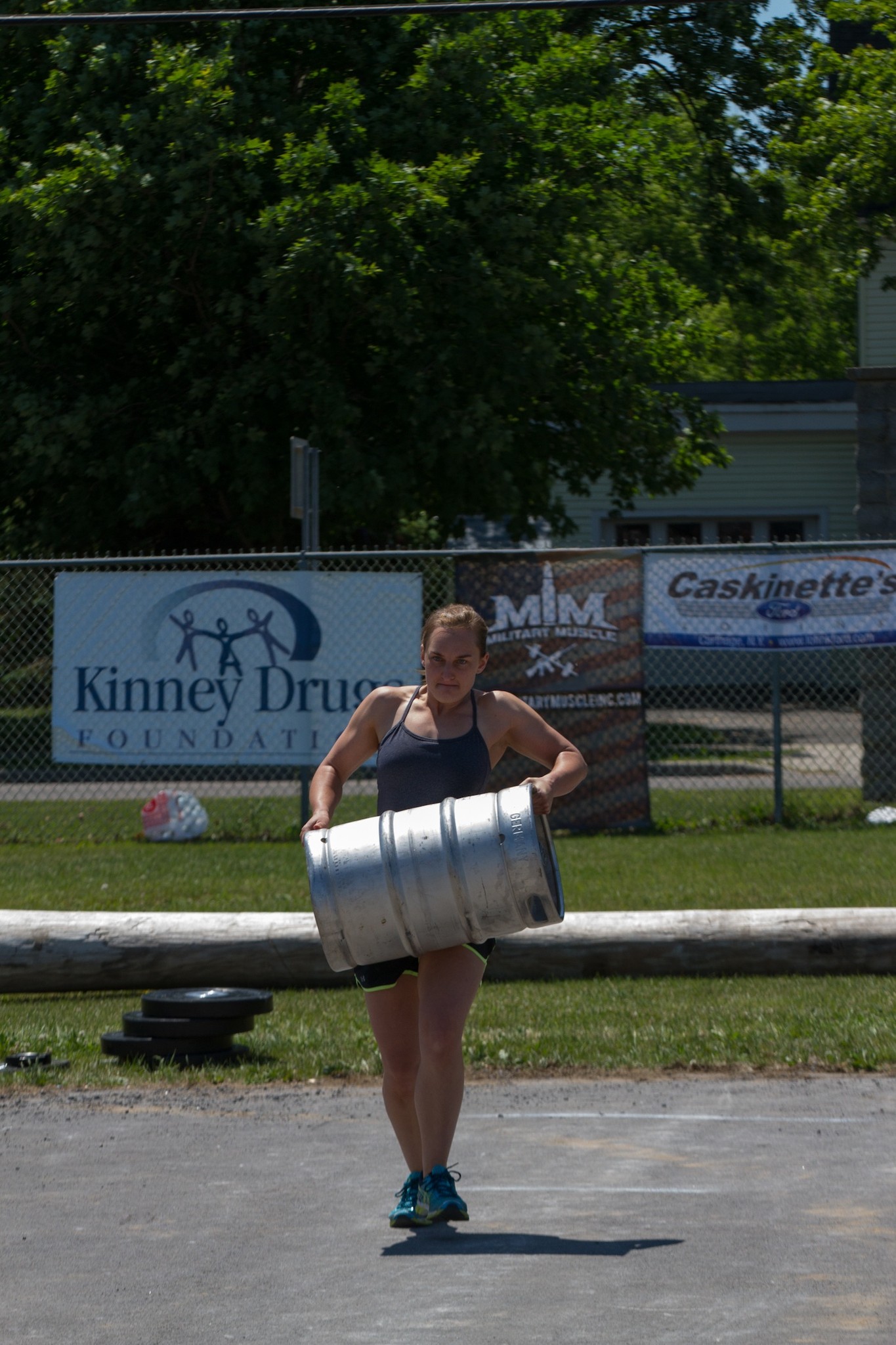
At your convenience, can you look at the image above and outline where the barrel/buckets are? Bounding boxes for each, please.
[303,783,566,974]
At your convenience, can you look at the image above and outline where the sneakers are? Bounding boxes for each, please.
[388,1171,432,1226]
[414,1161,470,1221]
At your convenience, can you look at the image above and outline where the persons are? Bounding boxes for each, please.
[299,602,588,1228]
